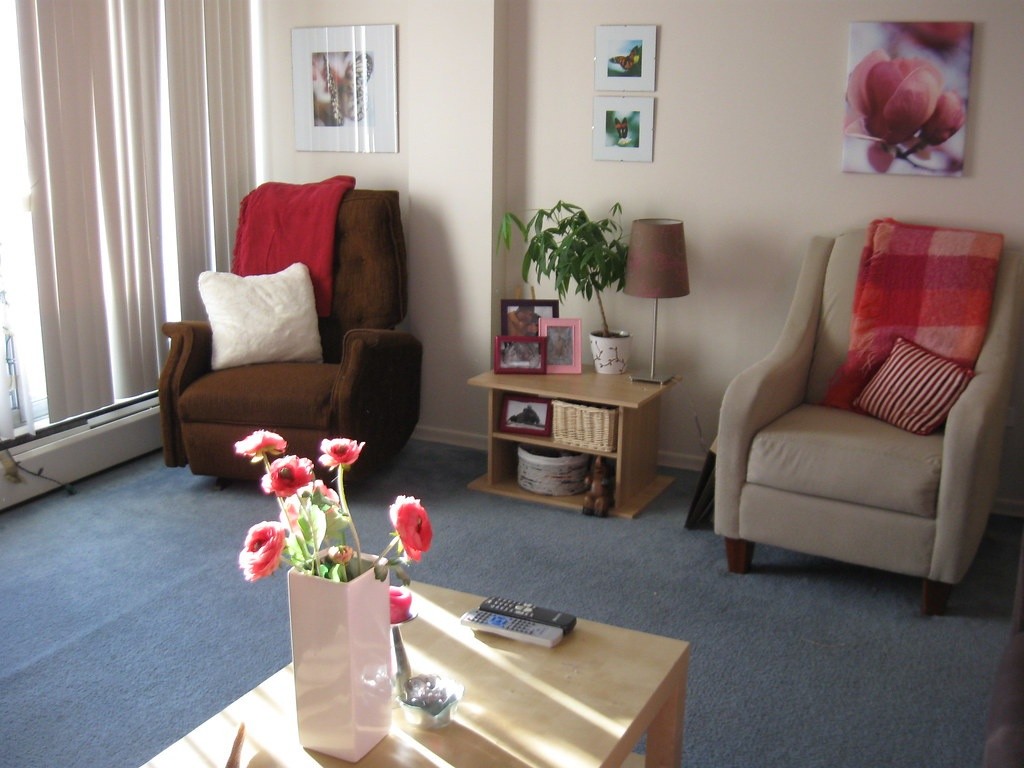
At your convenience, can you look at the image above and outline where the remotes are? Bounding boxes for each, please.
[460,610,563,647]
[478,595,577,634]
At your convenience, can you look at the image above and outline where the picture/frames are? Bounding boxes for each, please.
[496,392,553,435]
[494,299,583,376]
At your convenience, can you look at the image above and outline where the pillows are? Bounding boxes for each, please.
[197,262,323,372]
[852,335,977,435]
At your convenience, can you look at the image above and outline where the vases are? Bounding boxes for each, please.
[287,545,392,762]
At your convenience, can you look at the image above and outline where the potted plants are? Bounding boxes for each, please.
[495,200,632,375]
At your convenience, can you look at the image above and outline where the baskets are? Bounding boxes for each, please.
[552,399,619,454]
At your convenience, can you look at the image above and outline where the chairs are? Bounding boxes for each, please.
[714,219,1024,616]
[158,174,422,490]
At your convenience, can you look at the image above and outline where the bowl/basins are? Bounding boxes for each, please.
[396,677,458,727]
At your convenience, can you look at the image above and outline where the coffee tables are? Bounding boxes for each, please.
[137,579,691,768]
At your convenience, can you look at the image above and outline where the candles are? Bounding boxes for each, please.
[389,584,412,623]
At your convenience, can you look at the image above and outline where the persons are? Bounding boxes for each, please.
[582,455,615,518]
[507,306,541,336]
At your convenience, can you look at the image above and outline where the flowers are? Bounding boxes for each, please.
[233,428,433,588]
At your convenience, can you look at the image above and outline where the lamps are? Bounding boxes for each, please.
[623,218,692,385]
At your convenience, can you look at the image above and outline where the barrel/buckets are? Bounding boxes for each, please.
[517,443,590,497]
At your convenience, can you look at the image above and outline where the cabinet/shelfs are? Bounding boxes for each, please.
[467,368,683,519]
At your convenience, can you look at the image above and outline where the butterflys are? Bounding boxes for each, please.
[322,53,373,127]
[609,45,640,70]
[615,117,628,139]
